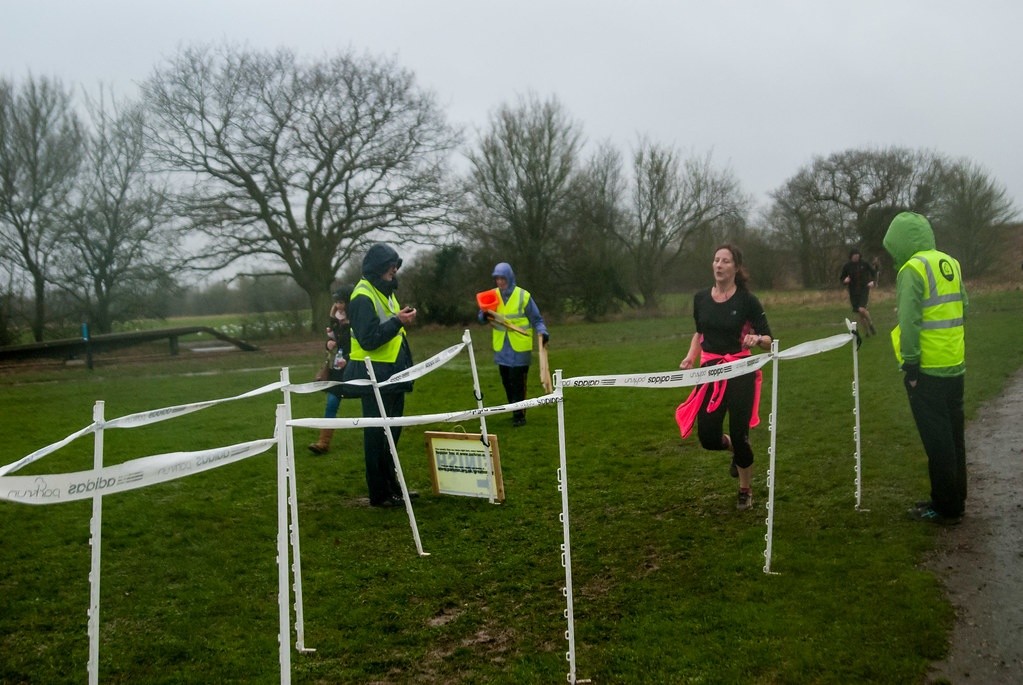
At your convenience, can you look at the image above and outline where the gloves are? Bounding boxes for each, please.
[543,334,549,342]
[482,312,495,320]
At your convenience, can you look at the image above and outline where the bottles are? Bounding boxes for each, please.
[327,327,336,341]
[333,347,343,370]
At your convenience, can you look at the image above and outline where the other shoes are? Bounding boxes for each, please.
[869,322,875,335]
[905,501,962,526]
[399,489,420,498]
[730,455,739,479]
[736,489,751,512]
[510,418,526,427]
[371,495,415,508]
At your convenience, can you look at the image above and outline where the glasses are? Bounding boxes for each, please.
[390,262,400,270]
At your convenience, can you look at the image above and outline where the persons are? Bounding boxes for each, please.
[883,212,968,522]
[680,244,773,511]
[339,243,421,505]
[841,249,877,337]
[308,284,355,453]
[477,262,549,424]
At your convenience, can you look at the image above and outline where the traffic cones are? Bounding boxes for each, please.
[476,290,499,323]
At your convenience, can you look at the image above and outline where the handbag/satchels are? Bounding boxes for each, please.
[316,352,332,393]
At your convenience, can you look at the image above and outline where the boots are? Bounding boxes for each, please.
[307,429,334,455]
